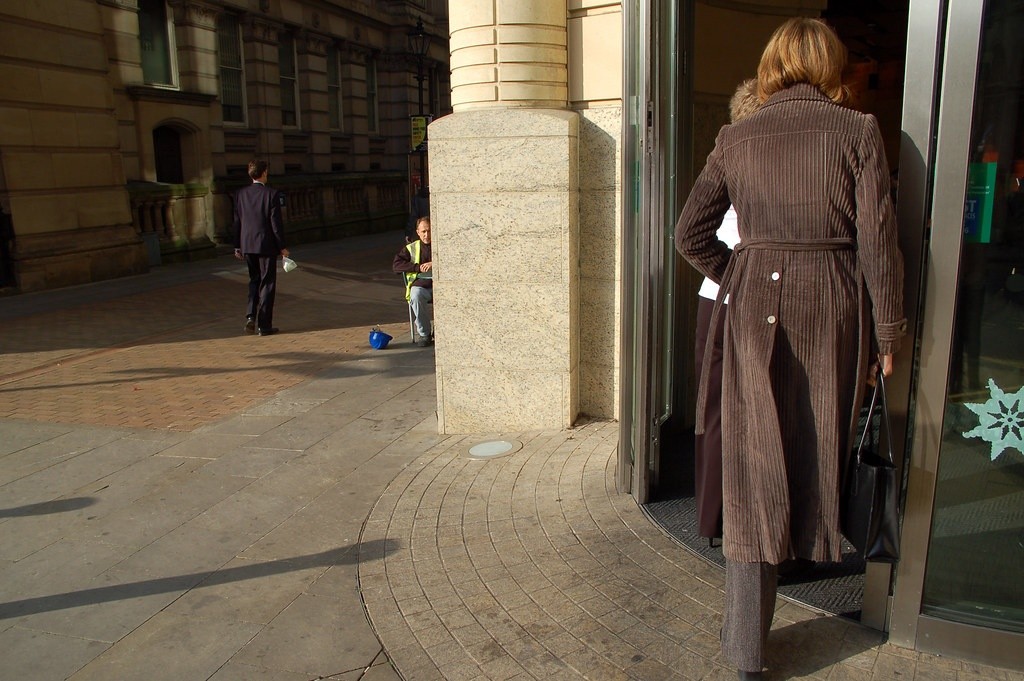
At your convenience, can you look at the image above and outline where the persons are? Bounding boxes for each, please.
[393,217,433,348]
[693,78,769,549]
[677,16,905,681]
[233,159,291,337]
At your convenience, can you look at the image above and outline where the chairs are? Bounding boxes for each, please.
[403,272,433,343]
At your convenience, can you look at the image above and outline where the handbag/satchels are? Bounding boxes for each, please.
[840,367,901,566]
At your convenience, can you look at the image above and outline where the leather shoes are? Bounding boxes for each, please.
[244,317,255,335]
[259,327,279,336]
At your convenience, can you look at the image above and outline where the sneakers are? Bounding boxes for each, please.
[417,332,432,347]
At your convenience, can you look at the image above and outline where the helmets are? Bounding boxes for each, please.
[369,331,393,349]
